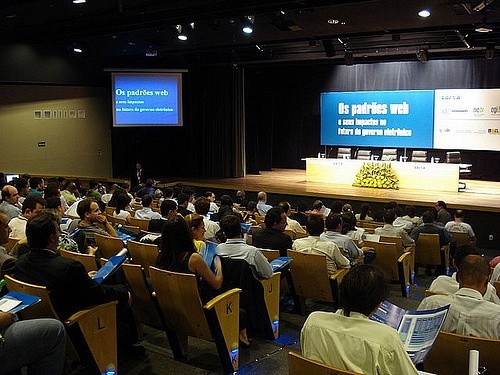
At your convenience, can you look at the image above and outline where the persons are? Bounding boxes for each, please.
[132,163,147,187]
[300,264,419,375]
[417,254,500,338]
[428,243,500,306]
[0,173,476,375]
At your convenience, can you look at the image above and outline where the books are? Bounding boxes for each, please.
[369,299,451,364]
[0,298,23,312]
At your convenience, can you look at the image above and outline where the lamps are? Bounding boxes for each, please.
[486,42,495,59]
[242,16,255,34]
[343,50,354,66]
[324,40,337,58]
[415,47,429,65]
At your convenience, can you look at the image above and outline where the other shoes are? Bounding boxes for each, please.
[120,346,146,359]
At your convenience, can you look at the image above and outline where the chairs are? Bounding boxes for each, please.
[0,198,500,375]
[357,150,371,159]
[337,148,352,158]
[381,149,398,161]
[446,151,463,164]
[412,151,428,162]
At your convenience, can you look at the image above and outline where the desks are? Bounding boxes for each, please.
[302,158,473,193]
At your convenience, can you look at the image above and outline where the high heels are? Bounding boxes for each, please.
[239,339,252,350]
